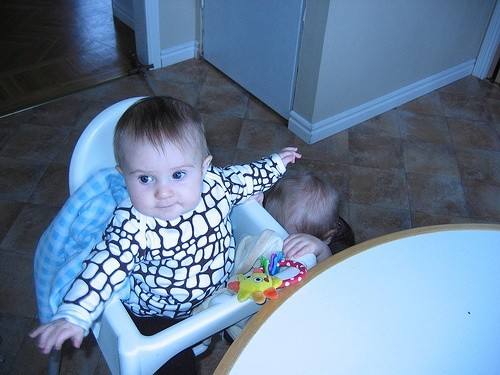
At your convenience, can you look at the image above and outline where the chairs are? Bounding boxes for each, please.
[48,96,318,375]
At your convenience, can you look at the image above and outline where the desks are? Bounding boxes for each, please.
[211,222,500,375]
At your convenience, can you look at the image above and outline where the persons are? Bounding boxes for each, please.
[29,95,302,375]
[256,165,357,266]
